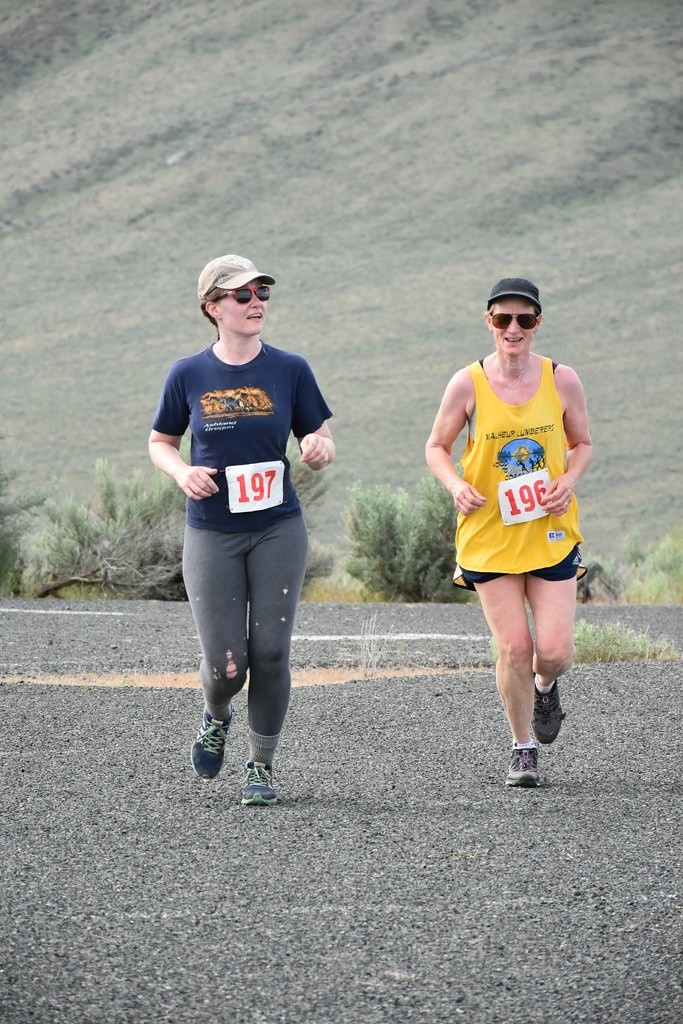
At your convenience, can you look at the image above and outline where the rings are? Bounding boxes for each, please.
[565,501,569,506]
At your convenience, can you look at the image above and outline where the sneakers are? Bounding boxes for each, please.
[507,743,540,787]
[531,673,562,744]
[242,761,277,805]
[190,700,233,779]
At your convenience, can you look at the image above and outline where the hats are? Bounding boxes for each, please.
[198,254,276,302]
[487,277,541,316]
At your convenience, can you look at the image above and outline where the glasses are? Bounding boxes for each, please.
[490,313,540,329]
[212,285,270,304]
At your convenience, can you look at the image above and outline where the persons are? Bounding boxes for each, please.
[148,254,335,804]
[425,278,592,787]
